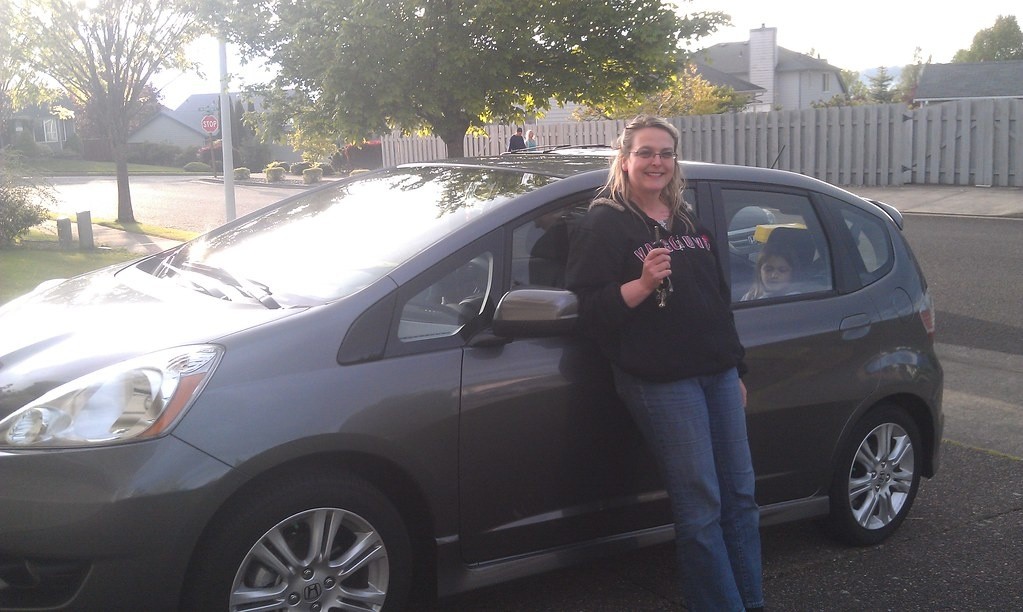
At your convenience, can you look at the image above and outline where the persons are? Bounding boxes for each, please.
[508,128,526,152]
[524,130,536,148]
[528,211,588,288]
[739,239,800,301]
[579,115,766,612]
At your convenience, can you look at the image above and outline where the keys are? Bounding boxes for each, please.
[656,275,673,307]
[652,226,665,248]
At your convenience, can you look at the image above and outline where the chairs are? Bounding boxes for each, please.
[508,217,570,291]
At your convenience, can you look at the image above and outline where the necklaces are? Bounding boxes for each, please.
[653,218,681,237]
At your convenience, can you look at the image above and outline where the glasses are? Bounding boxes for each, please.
[628,150,677,159]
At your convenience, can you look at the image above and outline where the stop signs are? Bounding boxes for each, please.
[202,116,218,133]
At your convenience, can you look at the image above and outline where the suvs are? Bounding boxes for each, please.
[0,143,949,612]
